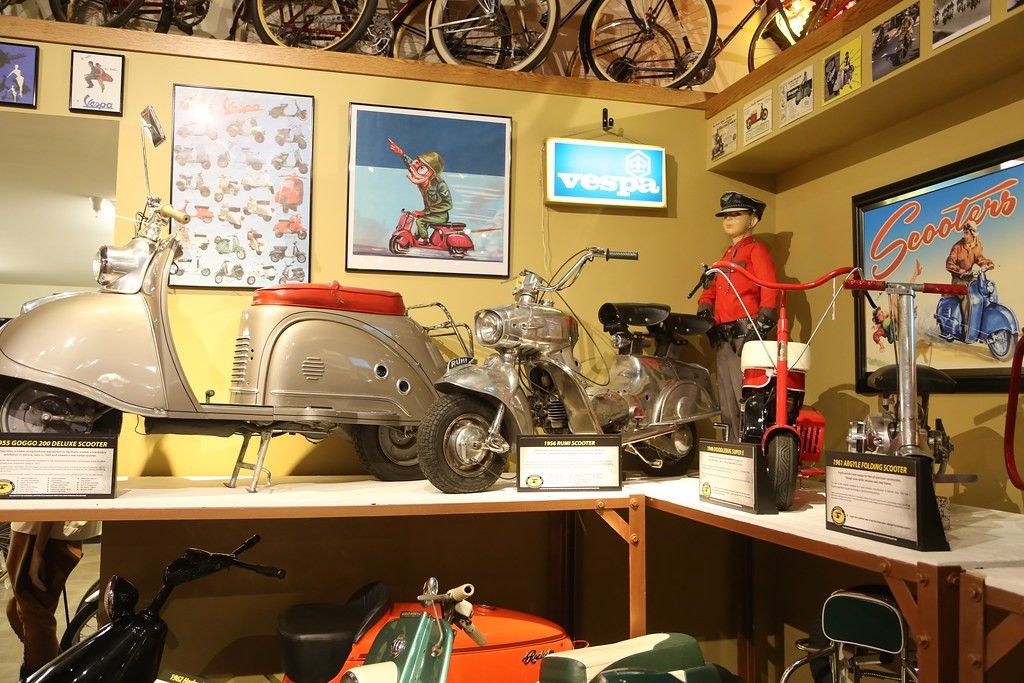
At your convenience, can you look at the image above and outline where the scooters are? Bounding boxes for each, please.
[795,80,812,106]
[15,532,906,683]
[746,101,769,130]
[710,131,725,158]
[933,0,980,26]
[833,64,855,96]
[174,87,309,286]
[0,101,477,496]
[416,245,722,497]
[686,263,872,508]
[840,273,978,532]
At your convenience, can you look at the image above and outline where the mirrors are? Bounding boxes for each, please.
[0,111,120,683]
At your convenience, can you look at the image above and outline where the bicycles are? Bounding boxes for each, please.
[45,0,847,88]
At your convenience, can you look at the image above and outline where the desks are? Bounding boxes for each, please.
[1,477,648,646]
[619,478,1024,682]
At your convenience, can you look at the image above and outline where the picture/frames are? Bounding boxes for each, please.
[850,137,1024,397]
[0,42,40,109]
[166,84,315,294]
[344,99,514,278]
[69,50,124,116]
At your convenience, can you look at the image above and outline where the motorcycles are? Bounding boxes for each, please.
[897,27,912,63]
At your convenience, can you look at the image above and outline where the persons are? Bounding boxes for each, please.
[697,190,778,442]
[5,520,103,680]
[897,11,914,51]
